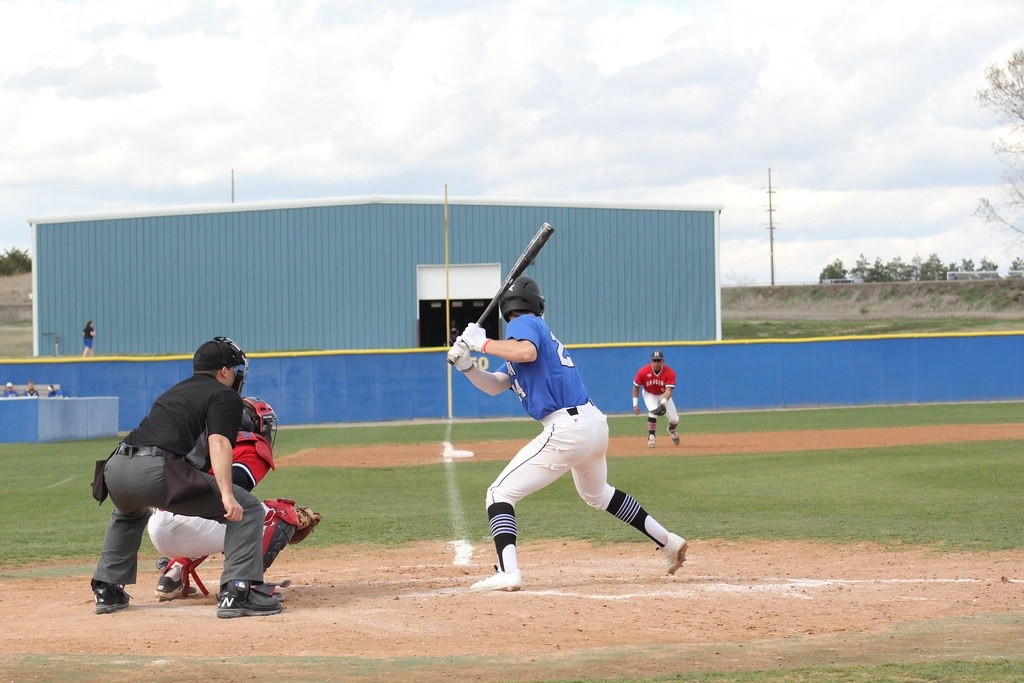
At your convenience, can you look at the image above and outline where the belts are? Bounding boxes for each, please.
[116,443,163,457]
[567,399,595,416]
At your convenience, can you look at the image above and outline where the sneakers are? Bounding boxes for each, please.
[93,584,133,614]
[469,567,522,594]
[155,575,197,600]
[250,583,284,602]
[655,532,689,577]
[216,579,281,619]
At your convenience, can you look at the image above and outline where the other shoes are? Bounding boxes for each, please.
[648,432,656,448]
[667,426,680,446]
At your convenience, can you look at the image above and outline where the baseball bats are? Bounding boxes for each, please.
[447,222,556,365]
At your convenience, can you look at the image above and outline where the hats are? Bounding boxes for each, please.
[650,350,664,360]
[193,340,240,372]
[6,382,13,387]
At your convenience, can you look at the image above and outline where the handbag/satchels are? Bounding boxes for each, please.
[91,447,122,506]
[163,458,215,508]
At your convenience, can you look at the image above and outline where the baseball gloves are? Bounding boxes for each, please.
[289,505,322,545]
[652,404,666,416]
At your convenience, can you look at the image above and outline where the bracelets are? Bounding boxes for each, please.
[633,398,638,407]
[661,398,667,405]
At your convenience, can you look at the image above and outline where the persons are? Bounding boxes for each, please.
[90,336,323,618]
[632,351,680,448]
[448,276,688,592]
[81,320,96,357]
[2,381,60,397]
[948,274,957,280]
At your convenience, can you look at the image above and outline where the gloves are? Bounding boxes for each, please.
[461,323,491,354]
[447,334,474,372]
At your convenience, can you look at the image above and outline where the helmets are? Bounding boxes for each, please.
[242,394,280,450]
[499,274,545,323]
